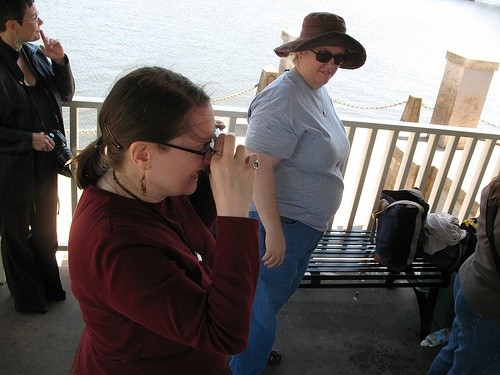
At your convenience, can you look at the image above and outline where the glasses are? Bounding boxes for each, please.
[5,12,39,23]
[311,49,346,65]
[149,136,211,161]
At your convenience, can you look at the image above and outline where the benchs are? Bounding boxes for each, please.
[295,229,450,341]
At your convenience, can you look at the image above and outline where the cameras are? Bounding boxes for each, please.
[49,129,75,165]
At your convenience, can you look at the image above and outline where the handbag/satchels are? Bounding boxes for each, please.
[371,187,429,268]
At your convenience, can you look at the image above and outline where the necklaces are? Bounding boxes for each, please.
[103,175,121,195]
[113,169,143,200]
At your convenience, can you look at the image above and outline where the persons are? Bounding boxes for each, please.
[424,172,500,375]
[68,66,261,375]
[0,1,75,314]
[230,12,367,375]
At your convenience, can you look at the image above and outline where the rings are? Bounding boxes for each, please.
[214,150,223,157]
[249,160,259,169]
[270,257,275,260]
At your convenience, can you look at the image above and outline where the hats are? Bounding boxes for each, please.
[273,12,367,69]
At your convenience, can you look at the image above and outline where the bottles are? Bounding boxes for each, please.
[420,327,451,347]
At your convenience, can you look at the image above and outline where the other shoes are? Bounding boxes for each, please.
[15,302,49,313]
[267,349,281,364]
